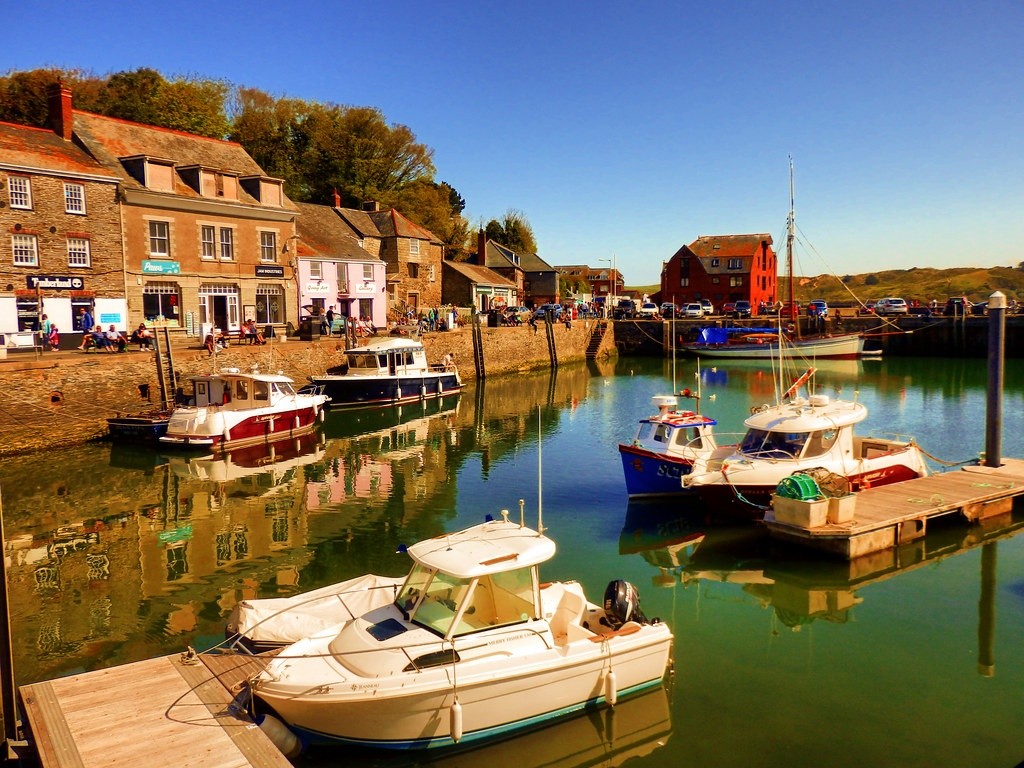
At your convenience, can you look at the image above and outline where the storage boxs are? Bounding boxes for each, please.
[825,493,856,524]
[769,492,829,529]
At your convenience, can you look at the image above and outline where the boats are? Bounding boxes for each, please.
[678,306,929,516]
[228,403,676,763]
[297,335,468,410]
[158,310,333,456]
[616,359,739,505]
[284,675,677,768]
[616,498,776,590]
[104,282,192,447]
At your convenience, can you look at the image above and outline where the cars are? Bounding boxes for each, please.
[970,301,1024,316]
[533,303,564,321]
[874,298,908,317]
[720,302,736,316]
[660,302,689,319]
[684,302,706,319]
[639,303,659,319]
[320,312,348,335]
[806,299,830,318]
[859,303,875,314]
[697,299,714,316]
[760,299,804,318]
[500,305,532,324]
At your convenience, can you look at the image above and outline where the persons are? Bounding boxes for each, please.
[319,308,327,335]
[130,323,156,352]
[443,353,453,372]
[529,315,538,333]
[41,314,59,352]
[326,306,334,337]
[560,300,604,332]
[1009,301,1016,316]
[77,308,128,354]
[203,328,228,356]
[347,315,378,339]
[501,311,523,327]
[452,306,459,323]
[835,309,842,324]
[417,305,447,334]
[241,319,266,345]
[397,315,408,335]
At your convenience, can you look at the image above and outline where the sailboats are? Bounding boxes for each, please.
[670,154,921,362]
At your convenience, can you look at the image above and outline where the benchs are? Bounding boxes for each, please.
[500,317,506,327]
[85,334,155,354]
[340,325,346,337]
[221,331,247,345]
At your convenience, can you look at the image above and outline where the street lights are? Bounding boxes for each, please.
[598,258,613,319]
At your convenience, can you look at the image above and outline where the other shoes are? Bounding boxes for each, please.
[255,342,258,345]
[250,343,253,345]
[89,346,95,350]
[117,350,124,353]
[113,350,118,354]
[262,340,267,345]
[140,348,145,352]
[108,351,112,354]
[76,346,84,350]
[144,348,152,352]
[51,348,59,352]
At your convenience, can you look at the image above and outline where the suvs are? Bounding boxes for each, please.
[732,300,752,319]
[614,300,637,319]
[941,297,968,316]
